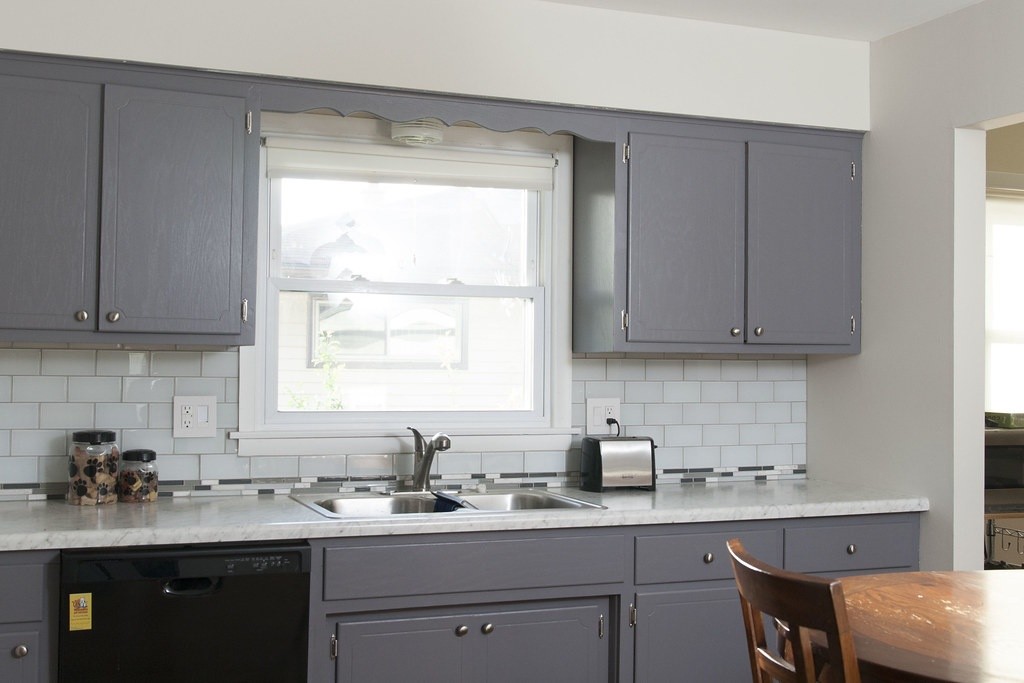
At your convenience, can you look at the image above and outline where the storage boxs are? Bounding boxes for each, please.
[985,411,1023,428]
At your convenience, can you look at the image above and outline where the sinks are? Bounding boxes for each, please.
[288,491,458,521]
[460,484,609,512]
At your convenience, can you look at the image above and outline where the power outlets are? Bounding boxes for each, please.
[584,397,620,435]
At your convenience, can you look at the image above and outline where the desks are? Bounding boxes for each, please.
[773,570,1024,683]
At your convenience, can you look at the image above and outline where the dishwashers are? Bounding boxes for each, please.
[56,541,311,683]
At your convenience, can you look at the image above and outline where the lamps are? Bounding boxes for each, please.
[391,117,442,145]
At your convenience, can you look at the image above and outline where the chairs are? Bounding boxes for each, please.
[726,537,862,683]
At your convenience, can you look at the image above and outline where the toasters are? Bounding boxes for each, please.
[581,436,655,492]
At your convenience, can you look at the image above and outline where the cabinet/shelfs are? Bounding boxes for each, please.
[631,511,919,683]
[0,48,258,346]
[0,564,44,683]
[574,104,862,353]
[328,604,603,683]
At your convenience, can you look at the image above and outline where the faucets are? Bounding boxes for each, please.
[405,425,452,491]
